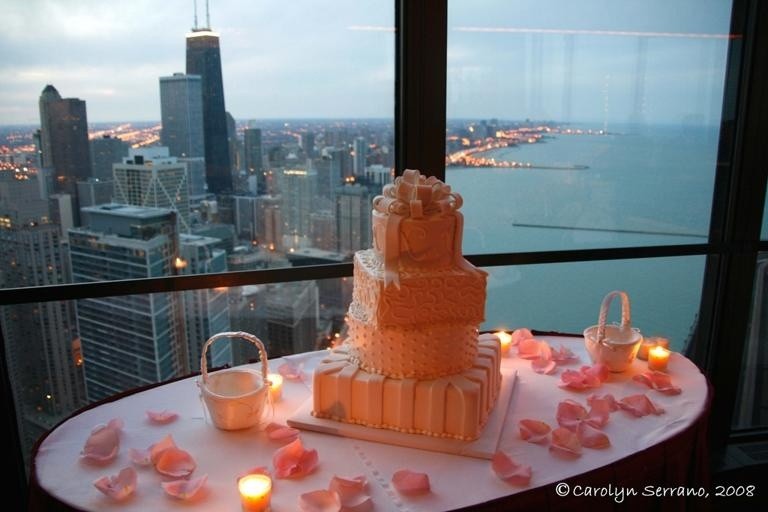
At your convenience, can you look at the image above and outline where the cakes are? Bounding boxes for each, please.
[310,169,502,441]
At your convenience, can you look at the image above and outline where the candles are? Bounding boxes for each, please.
[266,373,283,402]
[238,473,272,511]
[492,332,511,356]
[637,335,671,371]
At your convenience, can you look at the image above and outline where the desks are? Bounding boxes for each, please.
[30,330,713,511]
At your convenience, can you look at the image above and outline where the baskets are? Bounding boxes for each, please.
[199,332,271,430]
[584,292,642,371]
